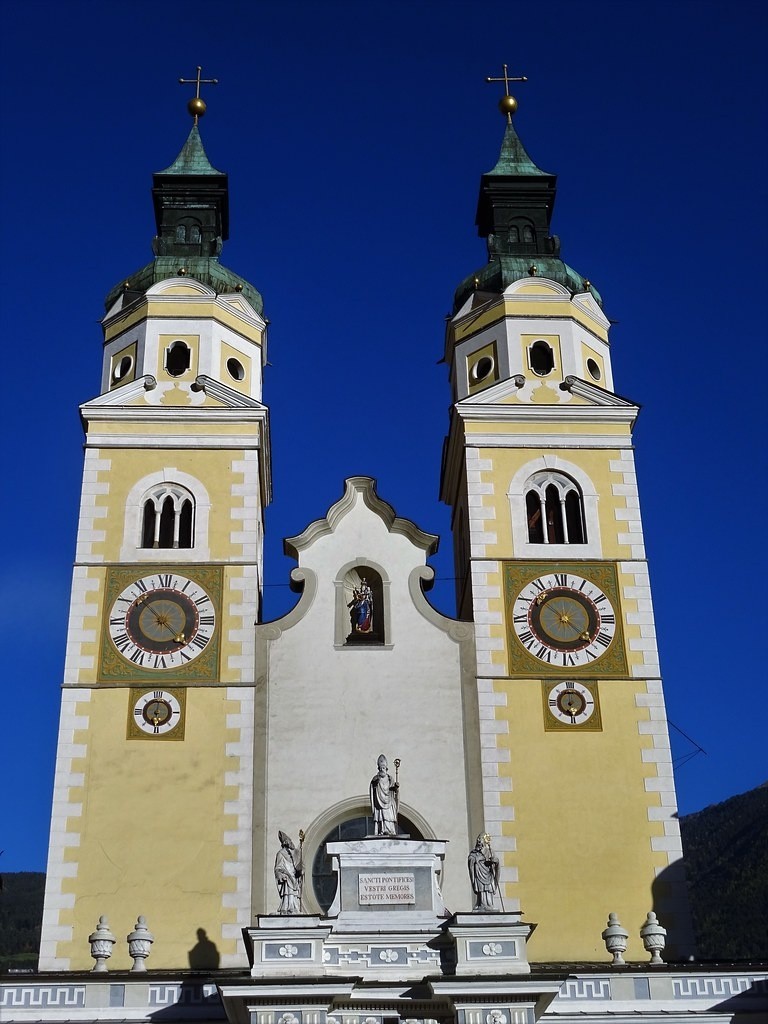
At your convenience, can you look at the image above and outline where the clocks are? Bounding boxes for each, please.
[511,572,618,672]
[132,690,183,736]
[546,681,595,726]
[105,570,218,672]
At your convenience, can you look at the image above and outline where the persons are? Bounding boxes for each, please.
[276,831,303,915]
[468,833,500,905]
[370,754,400,835]
[356,585,370,631]
